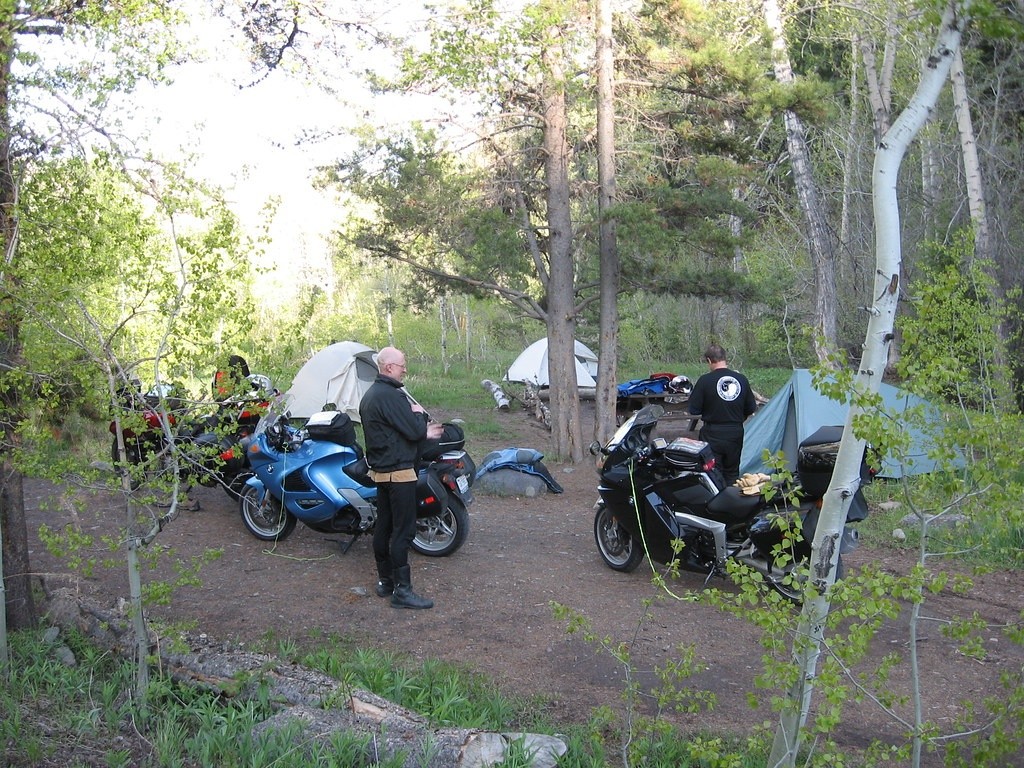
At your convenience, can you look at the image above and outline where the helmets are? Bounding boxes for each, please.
[232,373,275,405]
[669,376,693,394]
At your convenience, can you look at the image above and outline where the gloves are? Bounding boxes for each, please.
[740,487,763,496]
[732,473,768,487]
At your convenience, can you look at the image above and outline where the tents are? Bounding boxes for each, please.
[740,369,968,478]
[272,341,432,422]
[506,339,599,387]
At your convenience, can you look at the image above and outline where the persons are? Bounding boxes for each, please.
[687,344,759,487]
[359,347,444,609]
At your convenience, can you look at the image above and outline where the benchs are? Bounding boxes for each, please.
[658,415,701,420]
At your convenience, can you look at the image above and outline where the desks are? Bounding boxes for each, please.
[620,393,691,425]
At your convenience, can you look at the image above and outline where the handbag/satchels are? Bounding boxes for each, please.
[307,410,357,446]
[418,423,465,461]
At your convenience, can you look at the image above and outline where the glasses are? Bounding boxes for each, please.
[392,363,406,369]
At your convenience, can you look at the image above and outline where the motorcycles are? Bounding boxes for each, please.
[234,393,478,558]
[106,355,282,502]
[590,406,886,606]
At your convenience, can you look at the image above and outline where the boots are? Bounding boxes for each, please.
[375,560,394,597]
[389,564,434,610]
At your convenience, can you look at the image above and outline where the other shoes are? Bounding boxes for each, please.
[152,493,176,508]
[176,496,200,511]
